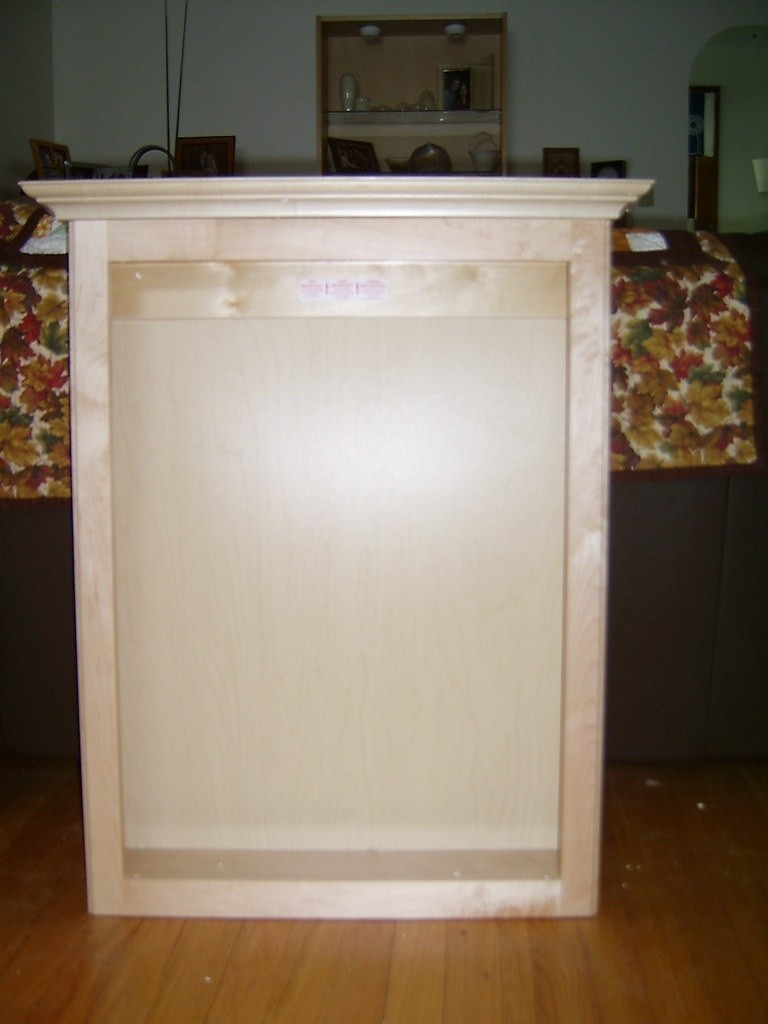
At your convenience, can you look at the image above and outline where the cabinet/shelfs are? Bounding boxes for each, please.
[24,172,661,909]
[314,9,510,176]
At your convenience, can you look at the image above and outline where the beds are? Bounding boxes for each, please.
[0,202,762,487]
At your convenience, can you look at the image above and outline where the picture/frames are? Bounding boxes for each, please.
[176,133,237,176]
[30,137,74,178]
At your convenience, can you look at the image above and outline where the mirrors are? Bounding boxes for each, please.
[685,23,768,276]
